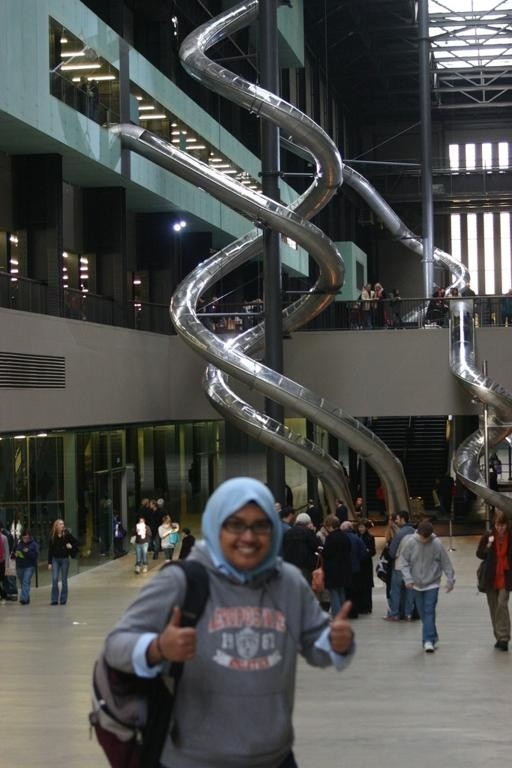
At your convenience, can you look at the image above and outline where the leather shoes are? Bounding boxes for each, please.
[499,640,508,649]
[383,614,401,621]
[50,601,57,605]
[406,613,413,622]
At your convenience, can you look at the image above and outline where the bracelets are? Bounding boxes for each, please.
[155,632,169,664]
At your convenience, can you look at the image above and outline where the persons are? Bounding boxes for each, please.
[424,285,476,328]
[76,75,100,120]
[348,282,403,330]
[239,298,264,330]
[197,296,219,331]
[276,459,455,653]
[0,518,79,605]
[97,495,196,575]
[102,475,356,768]
[487,452,503,490]
[501,290,511,328]
[474,510,512,651]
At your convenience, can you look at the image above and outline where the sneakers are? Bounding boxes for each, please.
[134,568,139,573]
[142,567,147,572]
[495,640,500,646]
[424,641,434,651]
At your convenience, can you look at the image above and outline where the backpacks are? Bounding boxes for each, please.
[87,559,209,768]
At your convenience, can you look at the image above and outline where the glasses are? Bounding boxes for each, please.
[223,520,274,535]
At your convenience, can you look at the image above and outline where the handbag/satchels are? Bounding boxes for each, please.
[477,560,487,592]
[4,554,16,575]
[312,557,325,592]
[376,550,388,581]
[169,530,180,544]
[70,546,79,558]
[129,534,137,544]
